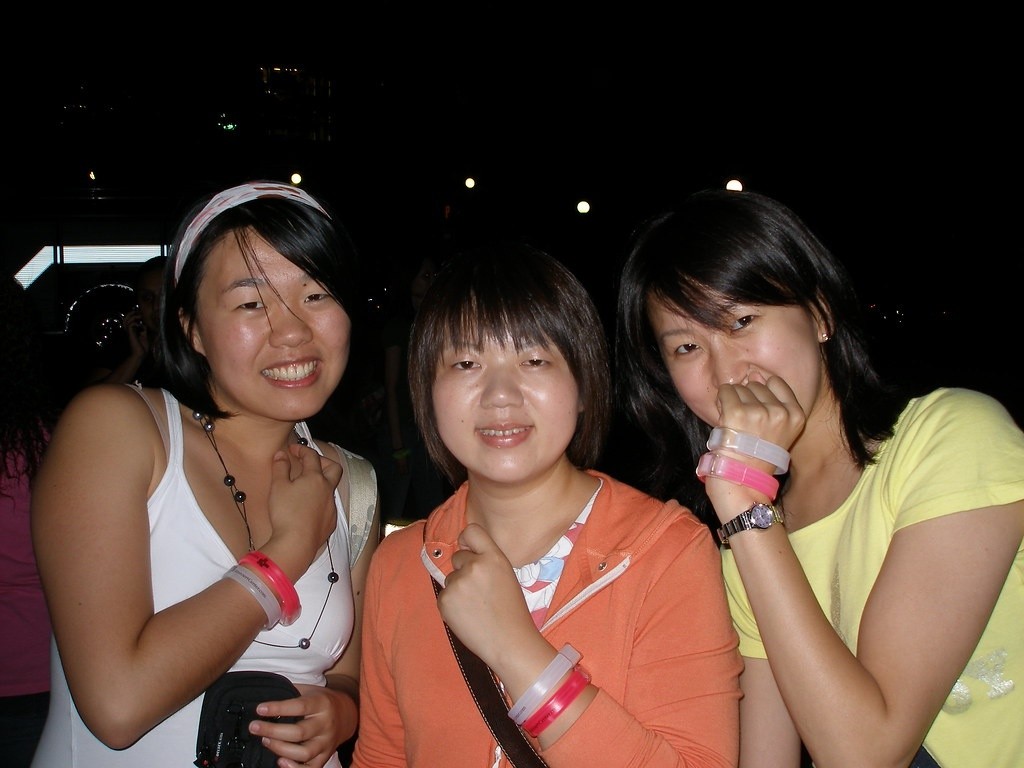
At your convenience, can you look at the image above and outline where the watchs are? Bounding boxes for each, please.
[716,501,784,544]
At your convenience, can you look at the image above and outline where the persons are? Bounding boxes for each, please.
[27,179,381,768]
[0,253,719,768]
[385,191,1023,768]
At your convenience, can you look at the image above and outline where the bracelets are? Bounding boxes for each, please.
[522,668,592,738]
[240,551,300,626]
[697,450,780,500]
[706,424,791,475]
[507,642,583,726]
[228,567,281,631]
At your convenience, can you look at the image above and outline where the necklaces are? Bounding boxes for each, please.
[193,408,338,649]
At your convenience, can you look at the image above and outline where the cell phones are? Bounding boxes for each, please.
[134,304,144,332]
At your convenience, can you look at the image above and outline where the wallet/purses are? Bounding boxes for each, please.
[194,671,304,767]
